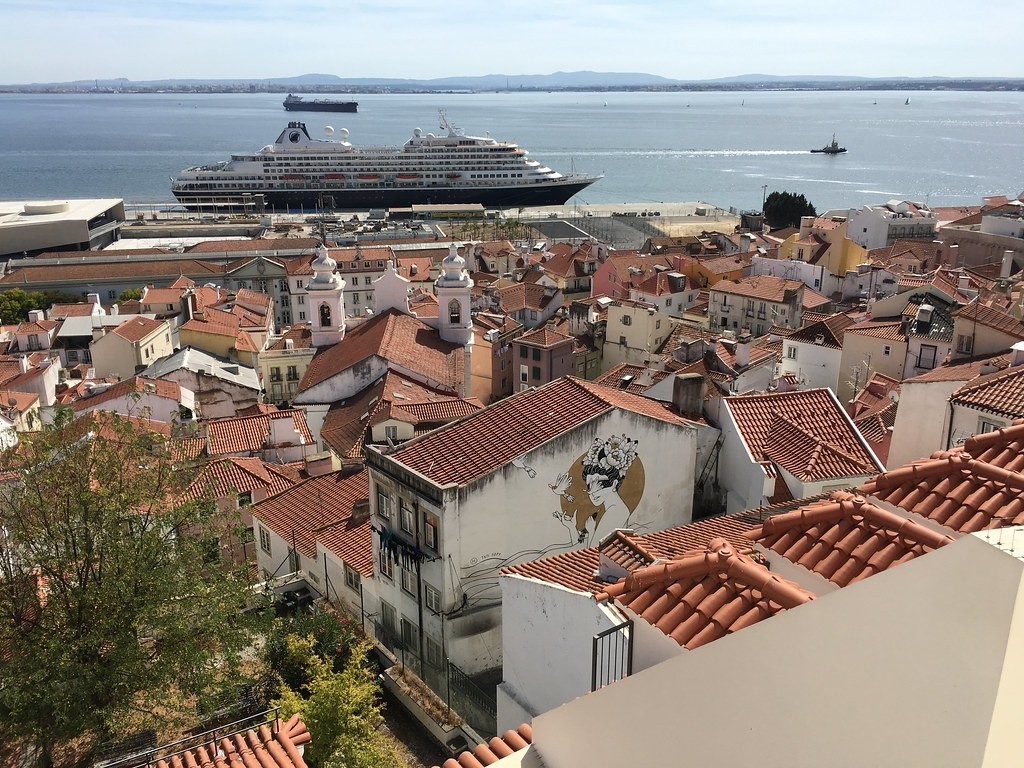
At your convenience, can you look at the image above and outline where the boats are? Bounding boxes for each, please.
[811,132,848,156]
[171,111,605,210]
[282,92,359,113]
[874,99,877,104]
[0,83,391,95]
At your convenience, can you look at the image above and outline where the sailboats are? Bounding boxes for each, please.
[904,97,912,105]
